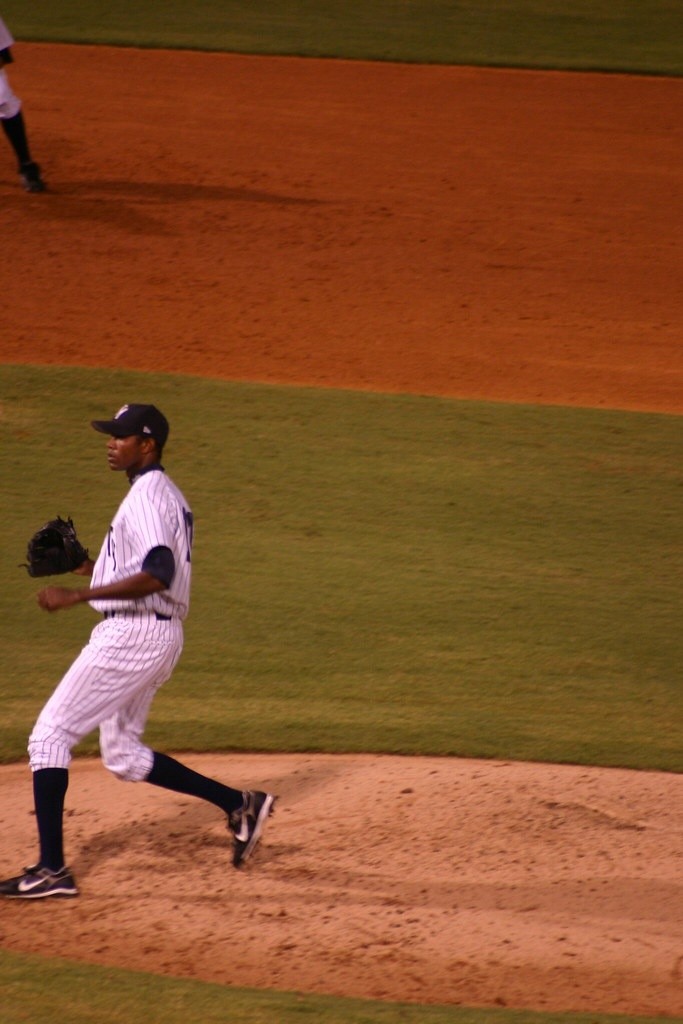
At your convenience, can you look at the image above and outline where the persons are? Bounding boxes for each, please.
[2,18,48,189]
[0,398,278,898]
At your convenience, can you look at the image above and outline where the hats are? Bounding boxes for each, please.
[91,403,171,445]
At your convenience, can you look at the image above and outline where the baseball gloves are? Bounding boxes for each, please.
[21,517,91,579]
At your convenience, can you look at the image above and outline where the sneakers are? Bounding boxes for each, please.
[228,789,274,869]
[0,864,80,898]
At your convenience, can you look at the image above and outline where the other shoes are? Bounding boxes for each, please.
[25,179,47,190]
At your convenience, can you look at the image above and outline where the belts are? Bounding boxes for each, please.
[102,610,173,620]
